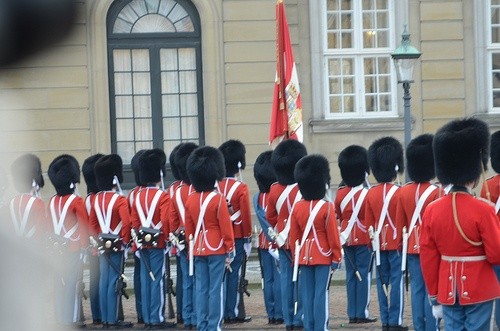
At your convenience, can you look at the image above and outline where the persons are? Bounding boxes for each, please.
[335,145,376,324]
[82,141,198,329]
[369,136,410,331]
[396,134,441,331]
[419,117,500,331]
[218,139,253,322]
[266,139,304,331]
[480,131,499,216]
[184,145,236,331]
[10,154,46,234]
[47,154,87,328]
[290,154,343,331]
[253,151,284,325]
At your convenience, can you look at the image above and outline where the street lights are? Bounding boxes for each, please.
[389,24,424,183]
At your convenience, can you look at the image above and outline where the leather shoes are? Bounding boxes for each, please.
[75,317,410,331]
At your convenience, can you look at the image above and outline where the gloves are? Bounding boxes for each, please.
[80,253,87,263]
[134,251,141,259]
[244,242,251,257]
[432,304,444,320]
[225,257,234,266]
[165,244,173,257]
[124,247,128,260]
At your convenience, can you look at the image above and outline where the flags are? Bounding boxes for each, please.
[269,2,303,143]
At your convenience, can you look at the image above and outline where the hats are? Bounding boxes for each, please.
[271,139,307,185]
[367,136,404,182]
[82,154,106,194]
[10,153,44,194]
[48,155,80,196]
[338,144,370,187]
[94,154,124,190]
[253,150,277,193]
[490,131,500,175]
[293,154,331,200]
[432,118,490,184]
[139,149,166,182]
[406,133,436,183]
[169,140,246,192]
[131,148,145,186]
